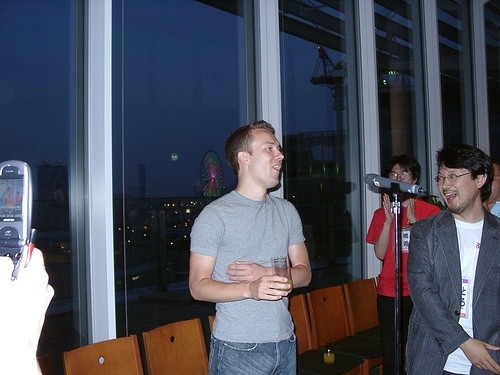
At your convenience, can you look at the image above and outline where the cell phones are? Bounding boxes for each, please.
[0,160,37,281]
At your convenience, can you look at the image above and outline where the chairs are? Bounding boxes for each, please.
[62,335,144,375]
[288,278,384,375]
[142,318,209,375]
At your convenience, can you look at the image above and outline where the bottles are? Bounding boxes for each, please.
[323,343,335,366]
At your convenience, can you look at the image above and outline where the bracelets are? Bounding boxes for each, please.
[409,220,417,226]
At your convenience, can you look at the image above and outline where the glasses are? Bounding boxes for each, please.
[389,172,411,181]
[434,173,471,182]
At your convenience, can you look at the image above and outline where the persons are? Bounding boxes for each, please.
[0,247,56,375]
[188,120,312,375]
[405,145,500,375]
[482,158,500,219]
[366,154,441,375]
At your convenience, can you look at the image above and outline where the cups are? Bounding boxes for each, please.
[274,256,293,293]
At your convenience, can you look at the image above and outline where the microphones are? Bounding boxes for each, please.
[364,174,423,195]
